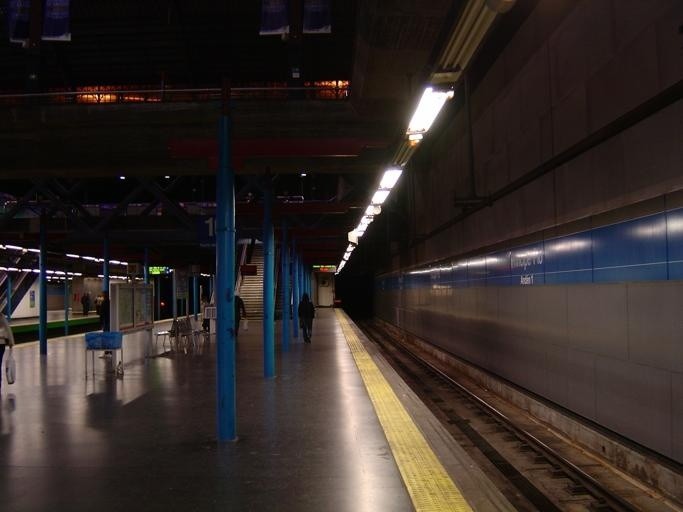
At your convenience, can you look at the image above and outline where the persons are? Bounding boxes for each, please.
[201,296,210,332]
[0,312,14,388]
[81,290,111,354]
[299,292,314,344]
[235,296,246,336]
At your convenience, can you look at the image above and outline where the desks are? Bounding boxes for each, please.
[152,314,216,355]
[84,348,125,384]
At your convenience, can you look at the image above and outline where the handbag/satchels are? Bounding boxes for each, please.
[4,357,17,386]
[242,318,249,330]
